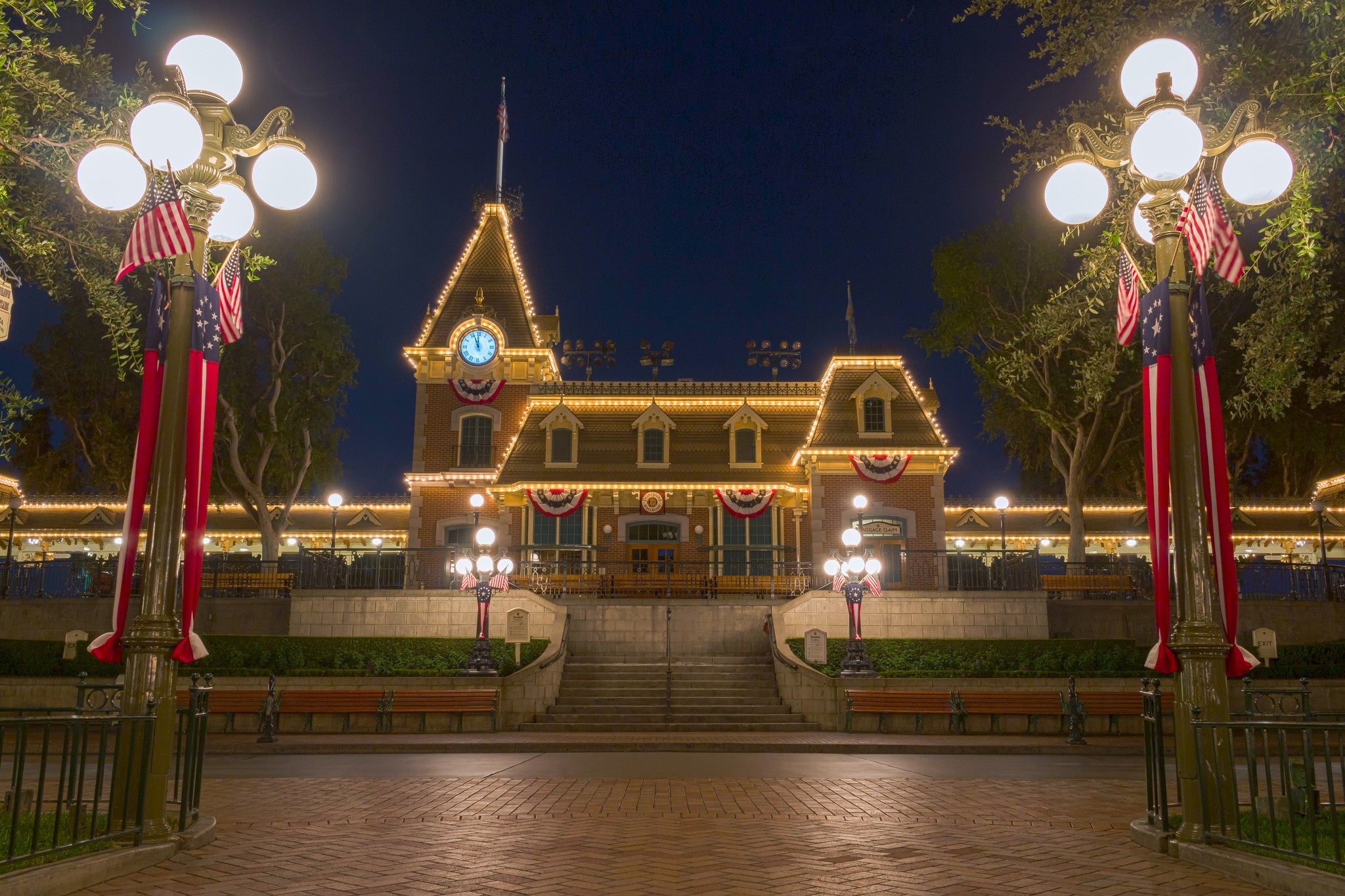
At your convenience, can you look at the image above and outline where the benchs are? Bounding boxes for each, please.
[1032,574,1140,600]
[200,573,294,596]
[509,574,813,599]
[844,690,1174,733]
[174,687,499,734]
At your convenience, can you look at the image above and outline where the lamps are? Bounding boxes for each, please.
[603,524,613,544]
[694,525,704,544]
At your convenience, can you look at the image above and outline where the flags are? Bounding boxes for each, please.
[1115,246,1140,346]
[488,567,509,593]
[202,243,208,282]
[497,83,508,143]
[210,245,243,347]
[832,568,846,592]
[460,567,478,590]
[863,567,881,597]
[114,172,194,284]
[845,284,857,346]
[1176,169,1244,284]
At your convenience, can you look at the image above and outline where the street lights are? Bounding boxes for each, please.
[2,497,22,600]
[78,34,320,856]
[470,493,484,576]
[853,495,867,581]
[456,528,513,677]
[326,494,342,590]
[1044,36,1294,844]
[995,496,1009,590]
[824,528,881,678]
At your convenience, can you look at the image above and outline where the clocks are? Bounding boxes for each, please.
[461,331,495,364]
